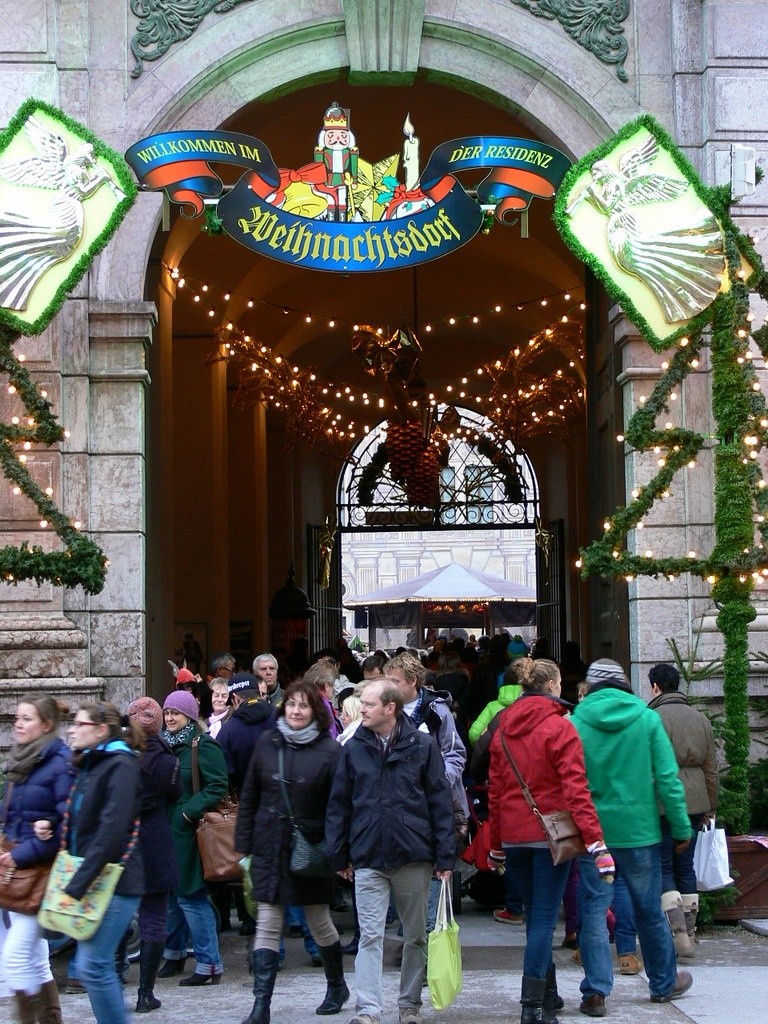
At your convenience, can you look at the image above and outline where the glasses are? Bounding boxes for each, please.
[327,659,337,666]
[68,720,99,728]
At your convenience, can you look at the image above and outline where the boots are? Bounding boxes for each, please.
[136,943,161,1013]
[317,940,349,1015]
[242,947,279,1024]
[520,964,564,1024]
[15,979,63,1024]
[661,890,699,964]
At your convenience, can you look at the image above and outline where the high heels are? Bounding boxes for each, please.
[158,958,187,977]
[179,972,221,987]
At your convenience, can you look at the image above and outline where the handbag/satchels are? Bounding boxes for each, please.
[0,836,50,915]
[39,849,125,940]
[427,879,462,1010]
[539,812,587,865]
[693,818,734,891]
[193,804,336,914]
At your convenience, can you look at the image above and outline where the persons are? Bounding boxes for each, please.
[469,669,526,908]
[33,701,147,1024]
[286,923,343,938]
[0,695,75,1023]
[114,697,184,1014]
[165,629,589,719]
[65,920,132,993]
[335,693,361,955]
[478,695,526,926]
[569,657,692,1016]
[386,899,403,936]
[383,651,471,988]
[561,868,644,974]
[646,661,718,965]
[275,905,324,972]
[312,648,357,910]
[211,673,268,936]
[157,691,227,985]
[234,680,350,1024]
[487,656,615,1024]
[326,680,457,1024]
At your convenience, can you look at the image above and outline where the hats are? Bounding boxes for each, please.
[586,658,625,684]
[506,635,527,658]
[129,671,258,741]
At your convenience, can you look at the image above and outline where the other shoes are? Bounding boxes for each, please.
[213,914,427,986]
[562,929,694,1017]
[66,980,85,994]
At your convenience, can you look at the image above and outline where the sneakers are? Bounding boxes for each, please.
[349,1013,381,1024]
[494,907,523,925]
[399,1007,424,1024]
